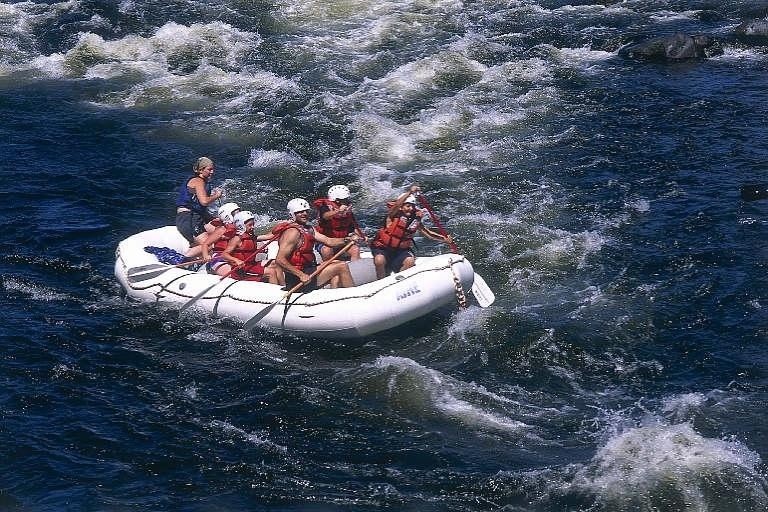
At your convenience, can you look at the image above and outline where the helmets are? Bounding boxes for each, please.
[233,210,255,235]
[217,202,240,225]
[397,192,416,205]
[327,184,351,202]
[286,197,311,223]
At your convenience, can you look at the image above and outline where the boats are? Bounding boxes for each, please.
[114,222,474,340]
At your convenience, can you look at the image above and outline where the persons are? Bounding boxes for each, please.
[220,210,286,287]
[313,184,369,263]
[271,197,360,293]
[368,185,454,280]
[200,202,241,278]
[174,156,223,259]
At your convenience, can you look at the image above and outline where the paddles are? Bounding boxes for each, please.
[128,255,222,283]
[243,242,354,331]
[416,190,496,308]
[180,234,280,312]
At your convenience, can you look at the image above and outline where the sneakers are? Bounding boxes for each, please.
[176,255,200,271]
